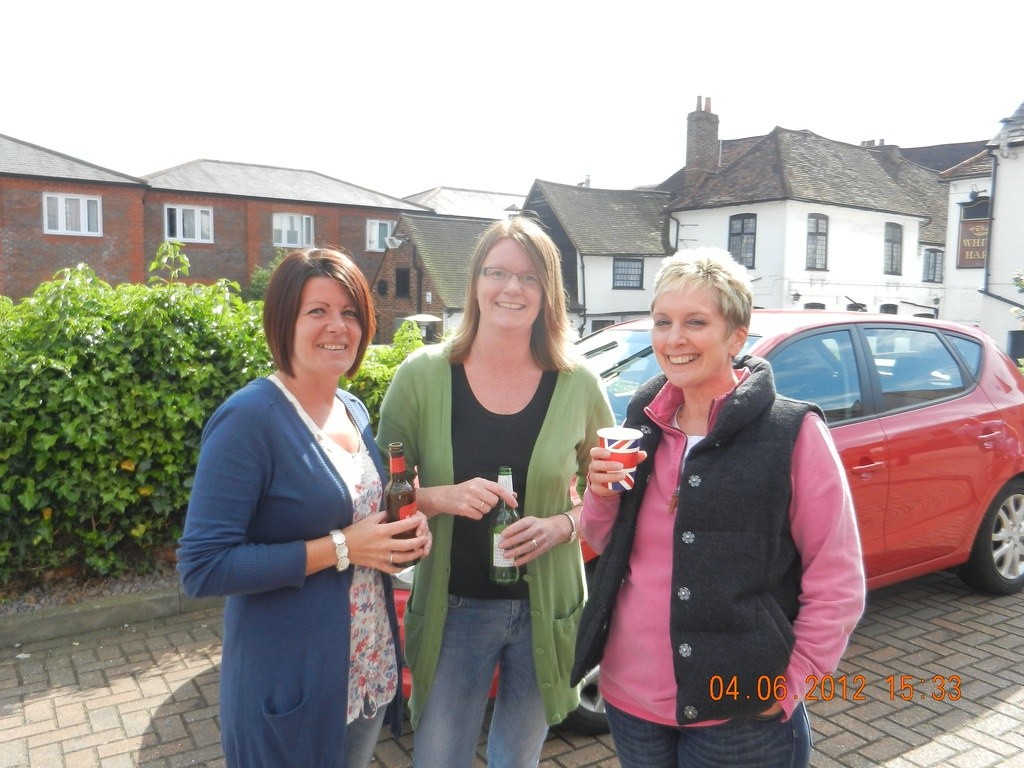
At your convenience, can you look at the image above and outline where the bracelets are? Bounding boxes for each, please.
[330,529,351,572]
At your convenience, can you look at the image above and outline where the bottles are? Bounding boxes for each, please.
[489,467,519,584]
[382,442,421,568]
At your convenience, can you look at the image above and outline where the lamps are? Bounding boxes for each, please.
[384,237,404,249]
[789,289,803,301]
[970,184,979,199]
[932,297,939,305]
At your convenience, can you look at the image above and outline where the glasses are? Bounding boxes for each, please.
[477,267,541,285]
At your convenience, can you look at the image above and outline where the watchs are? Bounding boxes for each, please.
[561,512,577,546]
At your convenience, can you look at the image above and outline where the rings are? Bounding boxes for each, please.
[479,500,485,511]
[529,539,538,549]
[389,551,394,565]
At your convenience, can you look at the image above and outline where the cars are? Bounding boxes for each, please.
[570,307,1024,602]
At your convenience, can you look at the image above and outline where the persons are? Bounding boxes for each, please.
[174,246,434,767]
[569,246,867,766]
[375,214,618,767]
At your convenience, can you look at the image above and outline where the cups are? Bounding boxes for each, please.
[596,427,643,493]
